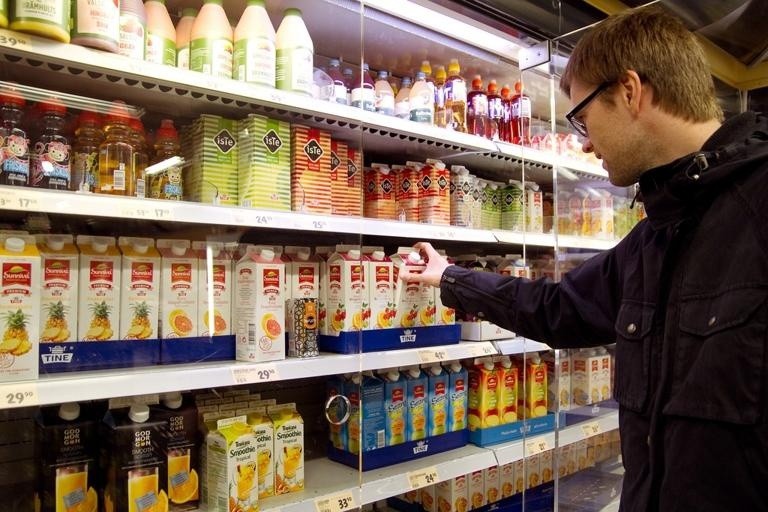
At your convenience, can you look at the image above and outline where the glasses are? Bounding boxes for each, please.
[566,72,616,138]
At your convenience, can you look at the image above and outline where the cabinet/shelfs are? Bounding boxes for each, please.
[1,32,634,511]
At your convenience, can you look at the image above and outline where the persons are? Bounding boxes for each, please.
[397,4,768,512]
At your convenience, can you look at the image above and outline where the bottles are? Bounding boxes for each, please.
[325,57,531,145]
[0,85,186,201]
[0,0,315,97]
